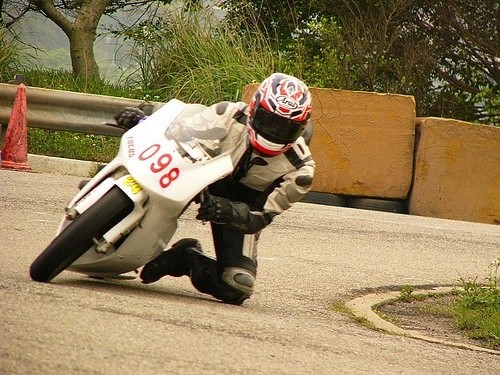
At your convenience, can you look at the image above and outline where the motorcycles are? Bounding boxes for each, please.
[29,98,236,283]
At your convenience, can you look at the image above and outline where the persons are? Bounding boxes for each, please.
[114,72,316,306]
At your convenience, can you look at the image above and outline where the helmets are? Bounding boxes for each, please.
[247,73,312,156]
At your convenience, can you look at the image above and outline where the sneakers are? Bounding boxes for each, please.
[79,180,90,190]
[140,238,202,284]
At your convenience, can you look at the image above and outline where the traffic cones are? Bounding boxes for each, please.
[0,82,33,172]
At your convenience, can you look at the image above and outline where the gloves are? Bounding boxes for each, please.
[114,106,145,130]
[195,196,249,230]
